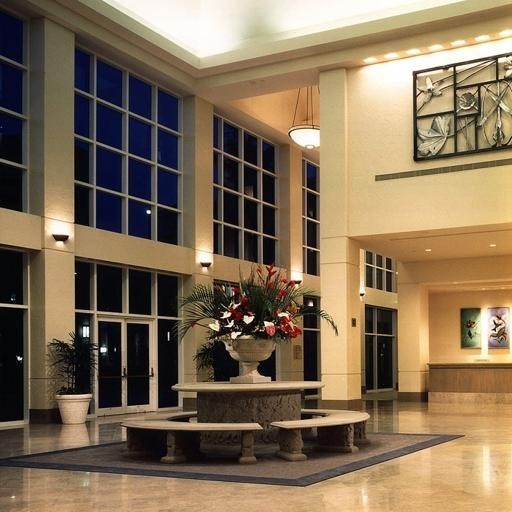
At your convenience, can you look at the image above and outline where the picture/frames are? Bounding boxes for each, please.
[460,307,509,349]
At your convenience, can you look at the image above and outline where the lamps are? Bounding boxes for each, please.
[52,222,69,241]
[287,84,320,150]
[360,290,365,296]
[294,275,302,284]
[200,256,211,267]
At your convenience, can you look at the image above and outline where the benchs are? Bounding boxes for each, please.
[120,411,263,463]
[270,408,370,462]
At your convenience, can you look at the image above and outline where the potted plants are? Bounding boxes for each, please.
[46,331,100,424]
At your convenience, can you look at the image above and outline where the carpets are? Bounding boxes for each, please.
[1,431,466,486]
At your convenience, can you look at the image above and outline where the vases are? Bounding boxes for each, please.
[208,333,285,384]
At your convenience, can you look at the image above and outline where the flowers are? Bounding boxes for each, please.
[171,263,338,343]
